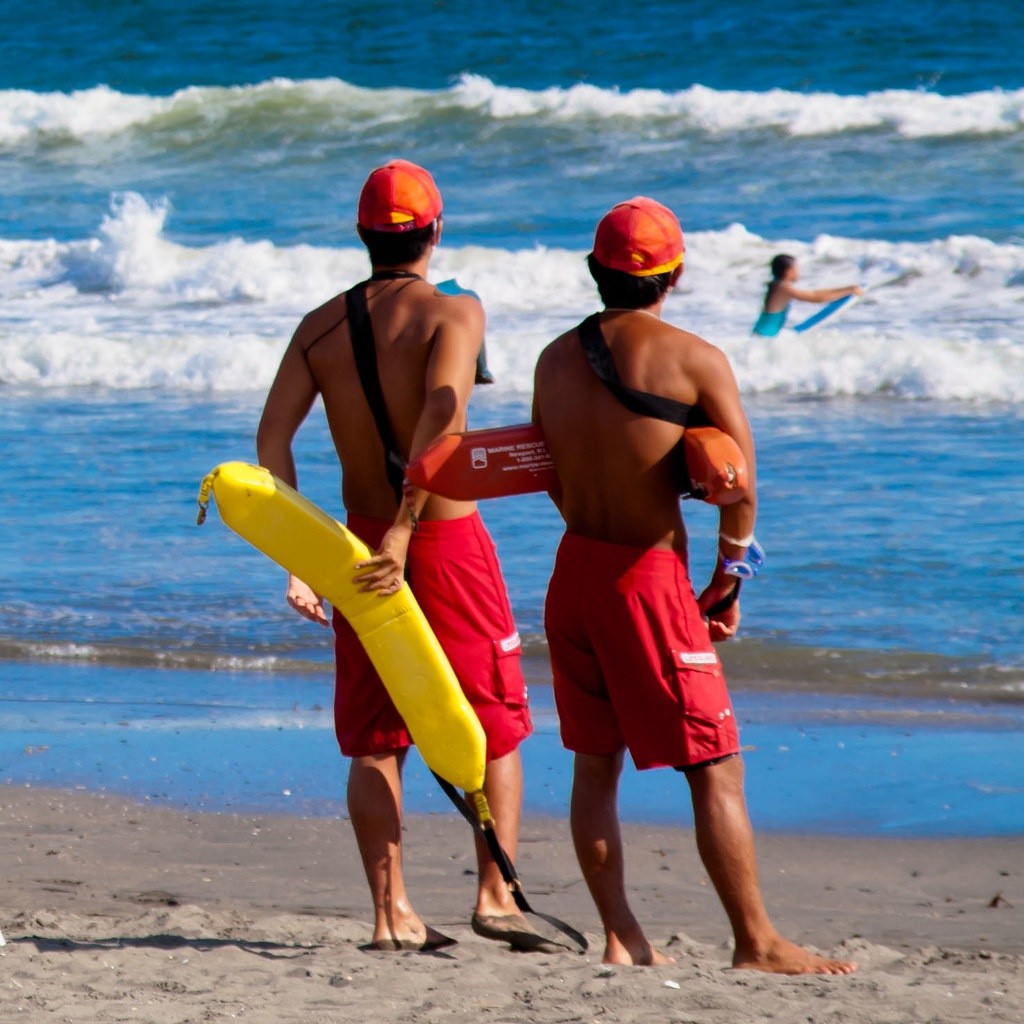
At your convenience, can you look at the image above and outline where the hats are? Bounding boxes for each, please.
[592,196,685,277]
[358,159,443,232]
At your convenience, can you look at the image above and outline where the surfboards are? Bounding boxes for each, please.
[792,282,874,333]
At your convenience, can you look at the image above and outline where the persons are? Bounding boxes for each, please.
[529,196,856,979]
[754,254,866,337]
[257,160,587,955]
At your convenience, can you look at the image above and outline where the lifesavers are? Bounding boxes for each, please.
[194,460,489,799]
[404,419,749,507]
